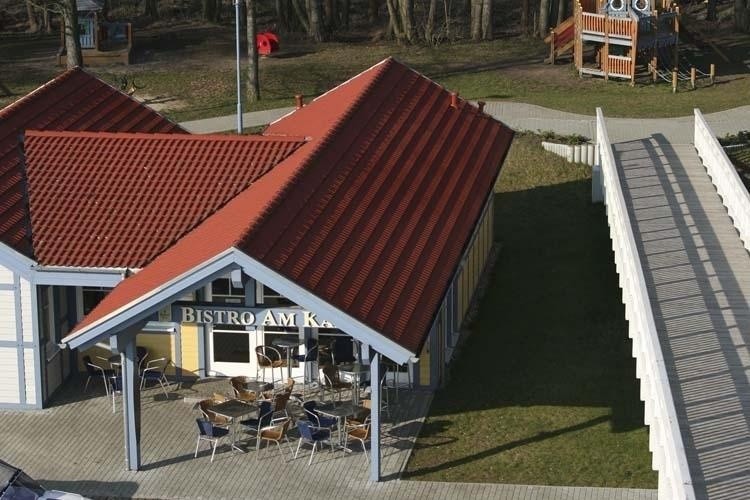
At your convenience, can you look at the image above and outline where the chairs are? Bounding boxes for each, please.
[82,336,408,467]
